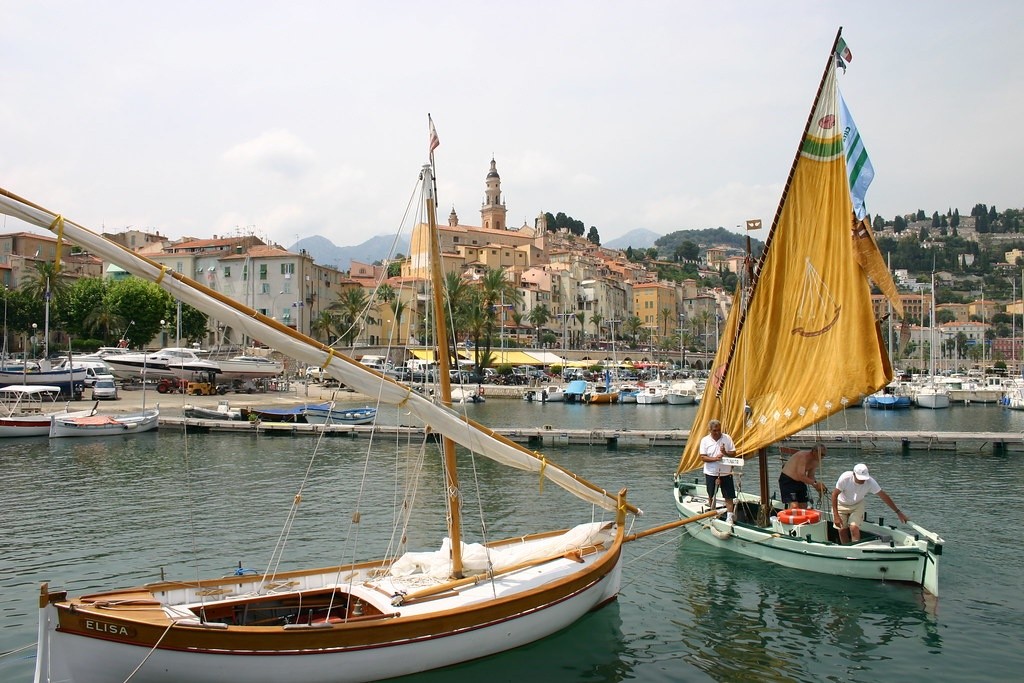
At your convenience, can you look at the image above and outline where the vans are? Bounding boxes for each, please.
[65,362,115,388]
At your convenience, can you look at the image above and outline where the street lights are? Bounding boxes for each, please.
[272,291,283,317]
[160,320,165,348]
[32,323,38,365]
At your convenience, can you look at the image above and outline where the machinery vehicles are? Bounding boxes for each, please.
[186,379,217,395]
[155,375,187,394]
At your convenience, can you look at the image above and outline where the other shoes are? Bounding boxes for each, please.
[726,518,734,524]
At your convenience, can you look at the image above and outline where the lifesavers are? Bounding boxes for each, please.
[777,509,821,525]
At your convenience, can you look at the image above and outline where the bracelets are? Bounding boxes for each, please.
[895,510,900,514]
[813,481,817,488]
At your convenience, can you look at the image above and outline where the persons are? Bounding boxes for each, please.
[832,464,910,545]
[699,419,736,526]
[776,443,827,512]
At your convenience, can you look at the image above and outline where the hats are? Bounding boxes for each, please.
[853,463,871,482]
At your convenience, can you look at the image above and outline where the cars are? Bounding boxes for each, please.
[563,368,710,381]
[92,380,117,401]
[305,355,551,384]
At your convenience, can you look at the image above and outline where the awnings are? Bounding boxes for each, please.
[406,347,566,366]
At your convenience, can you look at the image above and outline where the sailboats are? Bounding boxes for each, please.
[673,27,944,598]
[0,163,729,683]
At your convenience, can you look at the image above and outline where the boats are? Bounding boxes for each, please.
[48,344,160,436]
[182,400,241,421]
[241,401,336,421]
[300,406,376,424]
[0,385,100,437]
[522,252,1024,408]
[0,276,283,387]
